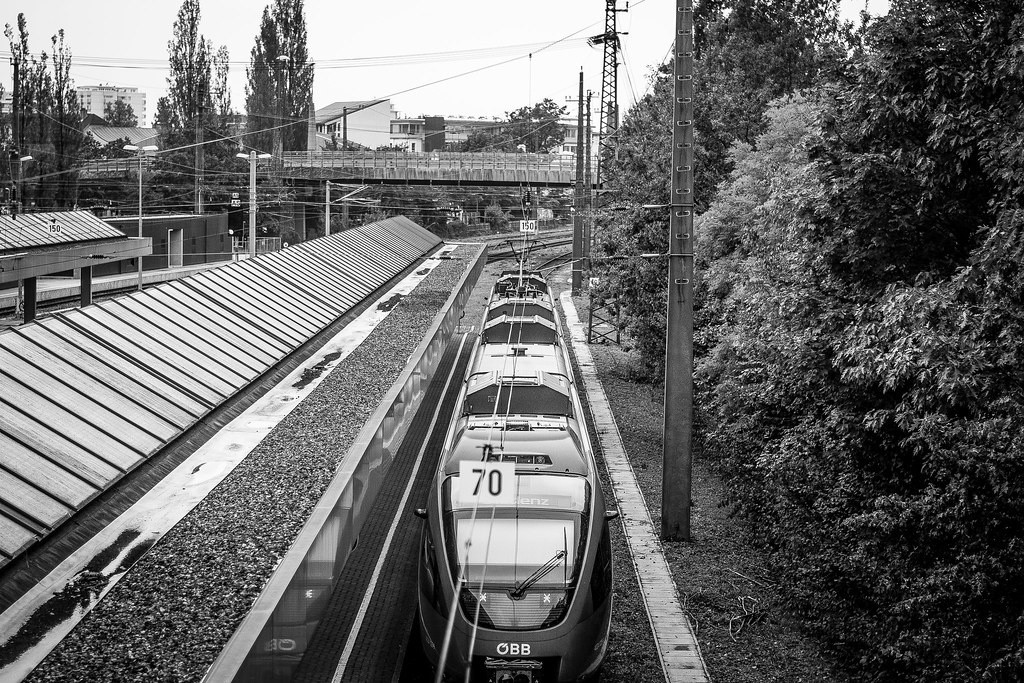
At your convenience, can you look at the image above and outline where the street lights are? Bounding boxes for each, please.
[17,156,33,214]
[123,144,158,289]
[236,151,272,258]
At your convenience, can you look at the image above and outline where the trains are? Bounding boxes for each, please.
[414,270,619,683]
